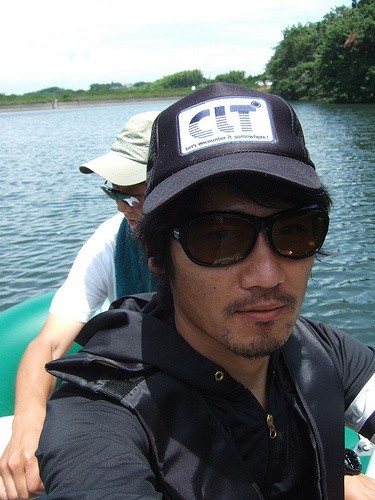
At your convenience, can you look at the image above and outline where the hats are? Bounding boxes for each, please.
[80,109,165,187]
[144,81,321,220]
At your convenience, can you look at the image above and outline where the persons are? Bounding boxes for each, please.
[31,82,373,499]
[0,107,169,499]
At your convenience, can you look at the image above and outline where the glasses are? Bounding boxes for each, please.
[99,180,146,207]
[164,206,330,268]
